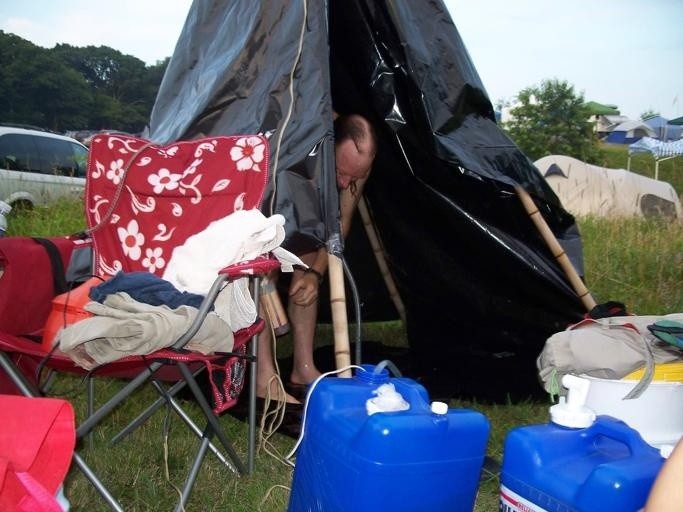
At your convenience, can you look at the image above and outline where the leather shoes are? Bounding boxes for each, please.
[256,395,307,418]
[285,379,314,397]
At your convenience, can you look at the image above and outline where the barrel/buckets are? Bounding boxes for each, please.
[498,413,666,511]
[548,373,683,460]
[286,364,490,511]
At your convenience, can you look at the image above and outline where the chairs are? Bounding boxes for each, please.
[1,131,286,512]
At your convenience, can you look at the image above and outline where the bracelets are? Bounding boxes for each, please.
[303,267,325,287]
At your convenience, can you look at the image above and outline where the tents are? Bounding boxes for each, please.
[529,153,683,223]
[580,100,683,161]
[140,0,589,436]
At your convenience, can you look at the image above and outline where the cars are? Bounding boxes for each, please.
[0,122,91,221]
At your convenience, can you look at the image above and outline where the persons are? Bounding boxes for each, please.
[246,109,377,406]
